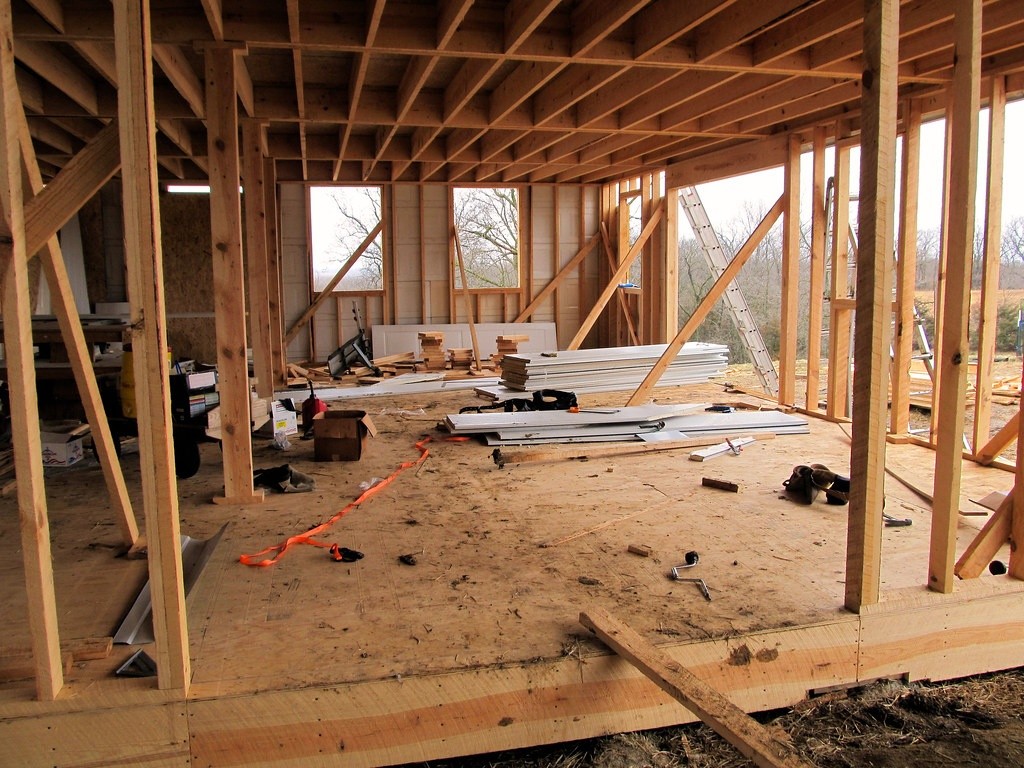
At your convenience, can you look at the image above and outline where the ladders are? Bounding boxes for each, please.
[817,177,973,453]
[676,185,780,398]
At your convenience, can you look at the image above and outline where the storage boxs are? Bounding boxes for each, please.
[186,371,215,389]
[312,409,377,462]
[189,392,220,418]
[252,397,297,438]
[41,430,92,468]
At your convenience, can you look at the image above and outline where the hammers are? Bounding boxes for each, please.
[882,511,913,528]
